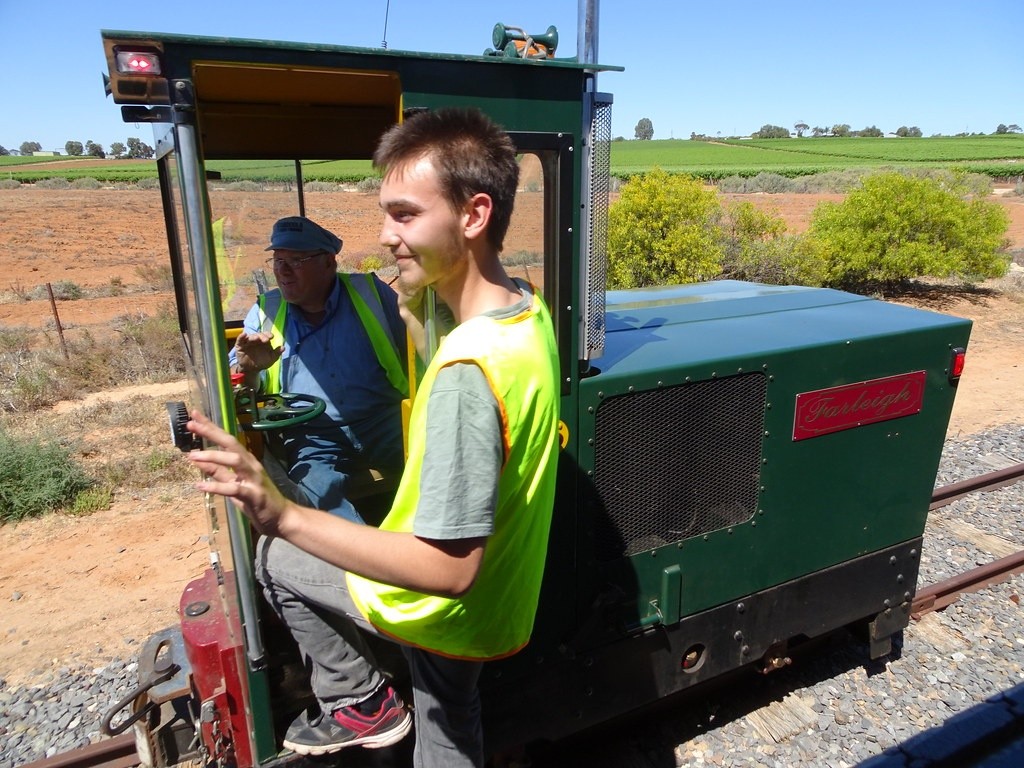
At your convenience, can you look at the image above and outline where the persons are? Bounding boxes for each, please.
[185,110,561,768]
[228,216,408,526]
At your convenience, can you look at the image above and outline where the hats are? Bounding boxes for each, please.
[265,216,342,256]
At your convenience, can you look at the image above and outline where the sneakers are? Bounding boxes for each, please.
[281,689,413,756]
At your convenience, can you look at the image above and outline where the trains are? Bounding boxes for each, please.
[92,23,976,768]
